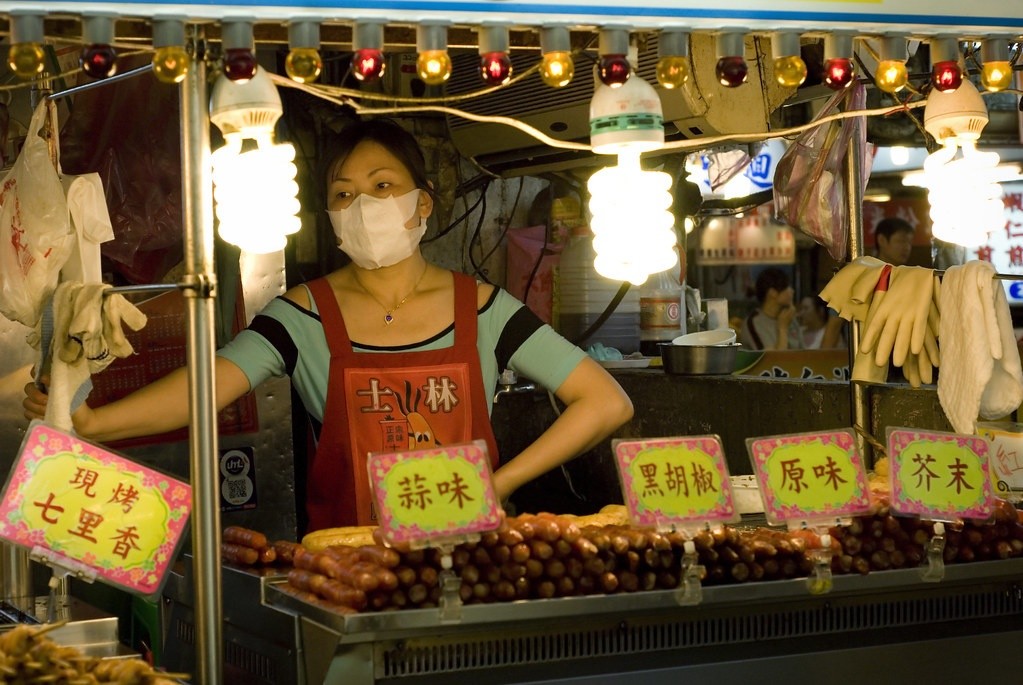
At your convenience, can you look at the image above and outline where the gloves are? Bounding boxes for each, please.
[52,280,146,371]
[818,254,943,387]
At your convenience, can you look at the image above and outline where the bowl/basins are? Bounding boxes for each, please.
[659,327,741,375]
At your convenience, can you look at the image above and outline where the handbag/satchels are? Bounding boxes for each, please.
[774,76,876,258]
[0,100,76,327]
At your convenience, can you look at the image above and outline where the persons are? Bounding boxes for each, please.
[818,218,916,383]
[739,268,807,350]
[23,113,635,533]
[796,294,847,349]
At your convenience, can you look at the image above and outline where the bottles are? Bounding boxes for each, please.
[557,225,681,358]
[0,540,32,598]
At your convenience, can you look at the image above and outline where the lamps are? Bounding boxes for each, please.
[922,44,1008,245]
[901,161,1023,188]
[209,37,302,254]
[586,32,680,285]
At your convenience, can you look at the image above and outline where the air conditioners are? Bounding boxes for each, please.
[447,27,773,181]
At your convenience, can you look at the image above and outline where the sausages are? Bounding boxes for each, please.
[218,488,1023,613]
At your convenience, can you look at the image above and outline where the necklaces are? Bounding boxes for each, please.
[352,258,427,325]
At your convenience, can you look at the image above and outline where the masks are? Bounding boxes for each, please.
[325,188,422,270]
